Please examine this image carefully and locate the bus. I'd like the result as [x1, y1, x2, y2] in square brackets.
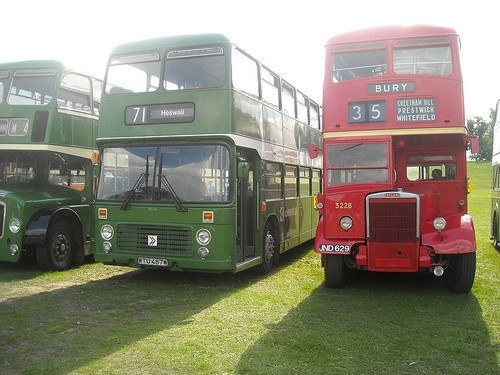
[0, 60, 115, 270]
[306, 25, 477, 294]
[92, 33, 324, 275]
[490, 99, 500, 249]
